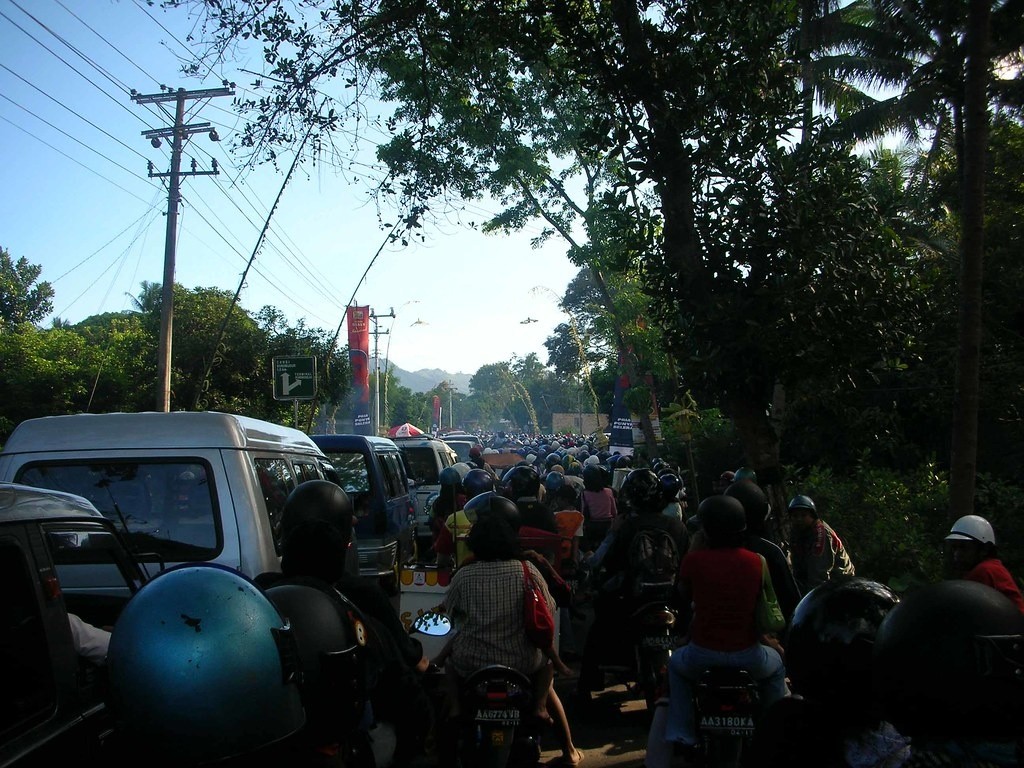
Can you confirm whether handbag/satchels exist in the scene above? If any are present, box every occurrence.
[521,561,555,649]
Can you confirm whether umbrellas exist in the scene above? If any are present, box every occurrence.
[388,422,424,437]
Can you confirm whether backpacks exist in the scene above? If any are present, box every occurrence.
[631,527,681,600]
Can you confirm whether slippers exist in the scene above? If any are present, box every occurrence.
[566,750,586,768]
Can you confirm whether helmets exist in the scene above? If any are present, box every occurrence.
[102,567,308,768]
[729,466,755,482]
[618,467,661,505]
[544,470,566,491]
[462,469,493,497]
[463,491,521,555]
[782,575,902,705]
[509,466,540,498]
[787,494,819,519]
[688,495,749,537]
[661,473,680,498]
[943,514,996,554]
[869,578,1024,738]
[272,477,359,567]
[450,433,687,475]
[438,467,461,486]
[259,581,377,750]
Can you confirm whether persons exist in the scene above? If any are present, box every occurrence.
[0,427,1024,768]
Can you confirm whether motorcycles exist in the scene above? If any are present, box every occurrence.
[592,566,684,730]
[676,665,760,768]
[447,665,553,768]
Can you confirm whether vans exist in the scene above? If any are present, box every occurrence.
[2,409,353,584]
[0,480,151,767]
[442,436,482,464]
[390,435,464,538]
[308,434,427,592]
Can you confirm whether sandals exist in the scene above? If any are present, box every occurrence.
[553,663,575,676]
[536,707,557,726]
